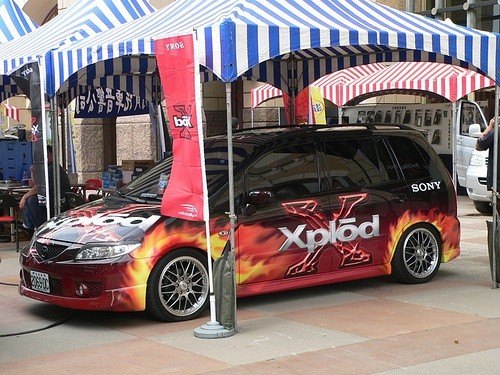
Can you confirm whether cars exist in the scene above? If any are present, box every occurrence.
[16,124,461,322]
[455,100,496,214]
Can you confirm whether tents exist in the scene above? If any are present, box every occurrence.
[0,0,500,122]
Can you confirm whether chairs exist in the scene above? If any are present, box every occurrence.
[0,178,119,253]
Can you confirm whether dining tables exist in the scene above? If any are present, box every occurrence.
[0,182,33,243]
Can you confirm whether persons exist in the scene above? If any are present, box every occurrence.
[476,99,500,221]
[17,145,71,233]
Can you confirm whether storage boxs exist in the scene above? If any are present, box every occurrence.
[102,160,153,192]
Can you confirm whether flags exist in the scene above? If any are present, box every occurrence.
[282,86,327,125]
[153,33,204,221]
